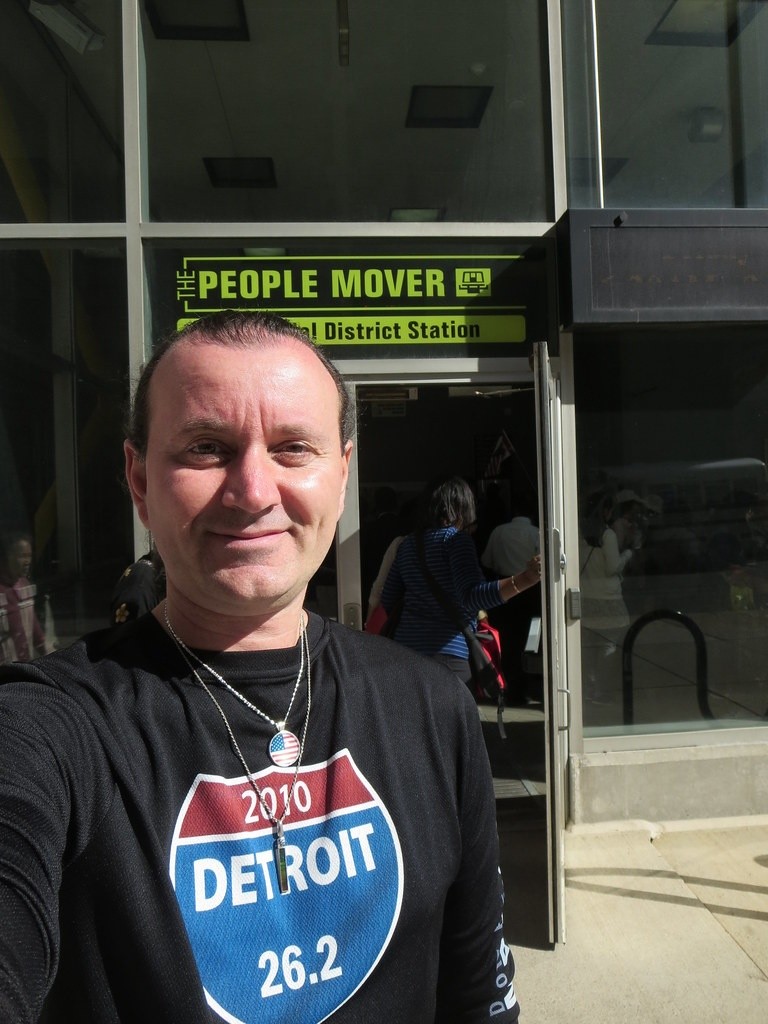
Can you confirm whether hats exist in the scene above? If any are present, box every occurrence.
[616,489,646,504]
[644,494,663,514]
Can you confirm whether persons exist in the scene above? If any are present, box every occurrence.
[580,490,659,706]
[1,312,542,1024]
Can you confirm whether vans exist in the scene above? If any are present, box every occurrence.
[577,457,768,573]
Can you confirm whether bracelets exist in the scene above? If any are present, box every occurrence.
[509,572,519,597]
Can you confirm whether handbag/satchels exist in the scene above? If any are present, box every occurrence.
[468,612,514,740]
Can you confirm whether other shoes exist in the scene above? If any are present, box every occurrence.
[585,692,594,700]
[592,695,617,706]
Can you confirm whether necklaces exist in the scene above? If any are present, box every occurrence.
[164,602,313,769]
[167,626,311,896]
